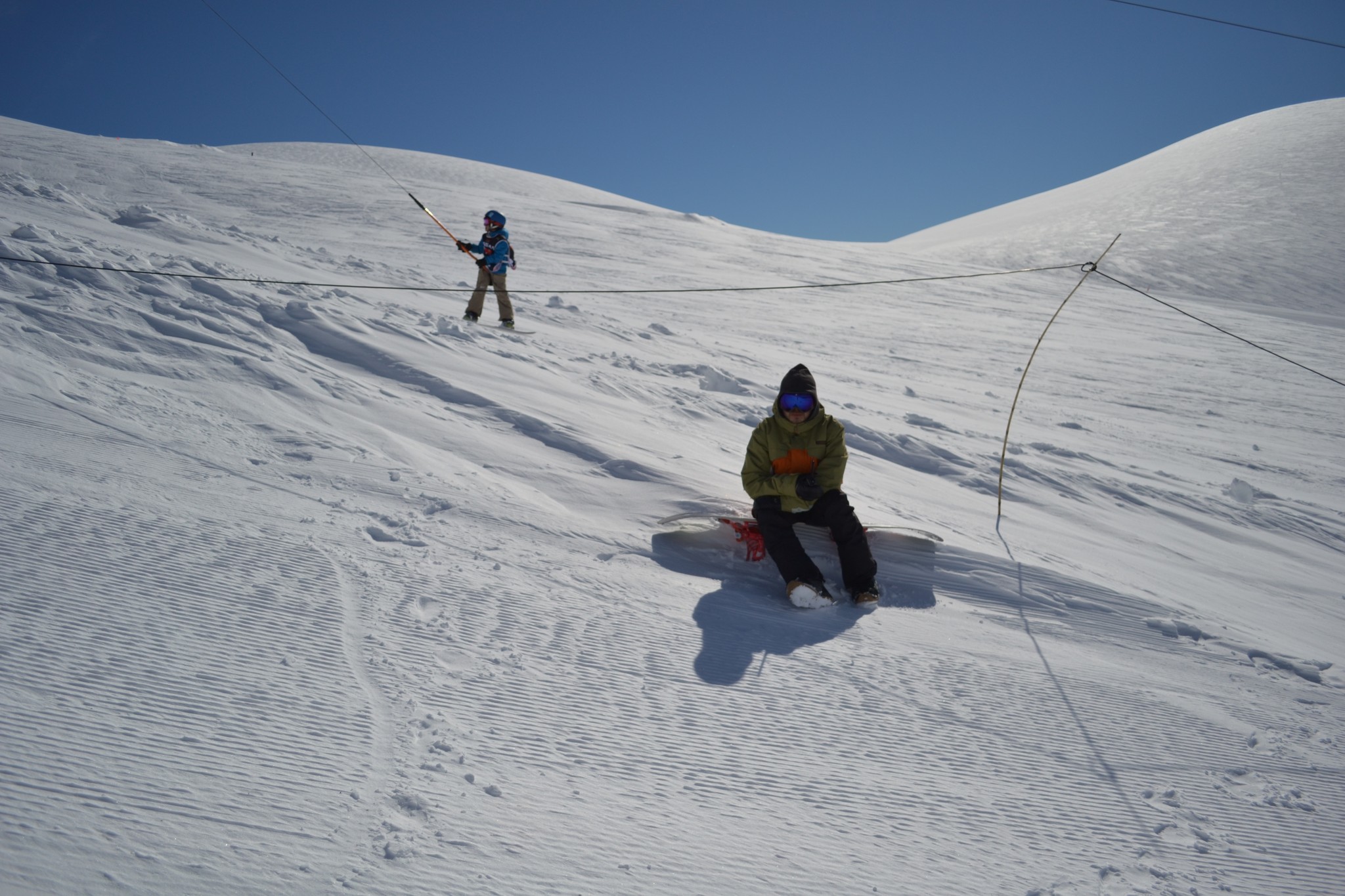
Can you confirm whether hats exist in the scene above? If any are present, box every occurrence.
[778,363,818,401]
[484,210,507,229]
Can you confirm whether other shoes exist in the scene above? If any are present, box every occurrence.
[498,319,516,330]
[463,311,478,323]
[787,576,836,608]
[852,576,882,605]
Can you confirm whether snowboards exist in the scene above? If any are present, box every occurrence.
[658,511,944,542]
[439,312,536,335]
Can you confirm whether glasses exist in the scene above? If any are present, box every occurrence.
[482,217,502,227]
[778,392,816,411]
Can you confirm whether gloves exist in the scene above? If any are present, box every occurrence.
[475,258,487,269]
[795,471,821,502]
[455,239,472,253]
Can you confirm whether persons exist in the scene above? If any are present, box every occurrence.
[741,364,879,609]
[457,211,515,330]
